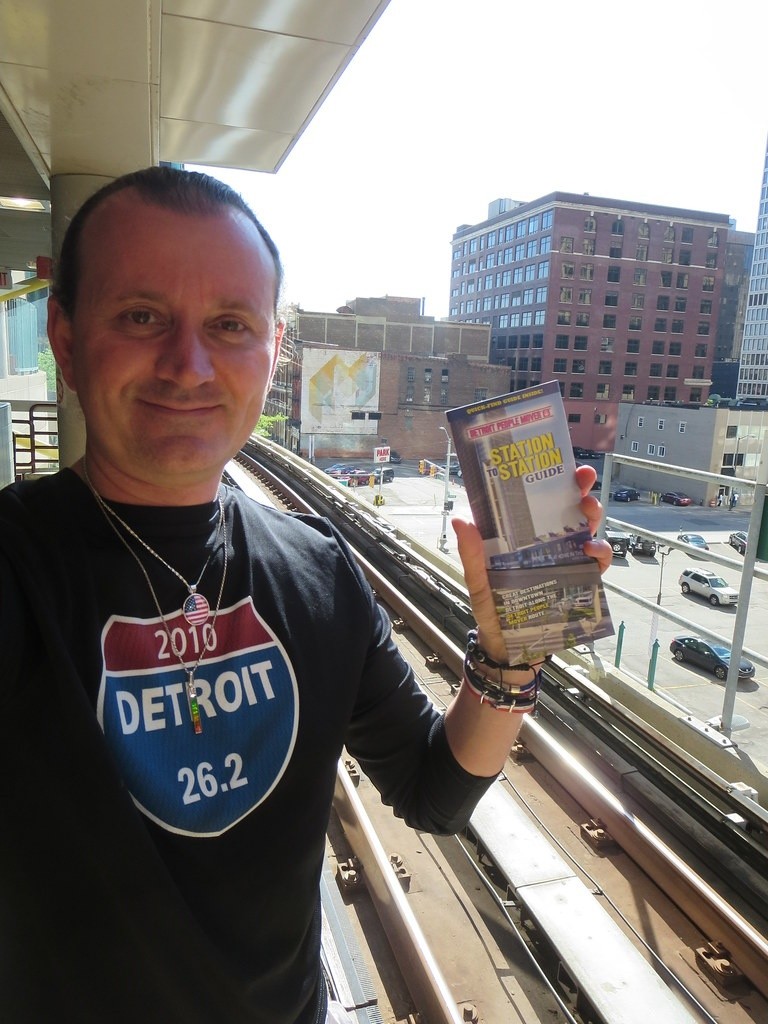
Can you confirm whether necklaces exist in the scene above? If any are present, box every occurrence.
[86,477,228,736]
[83,457,223,625]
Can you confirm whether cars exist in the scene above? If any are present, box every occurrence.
[325,462,370,485]
[441,463,463,478]
[670,636,755,679]
[614,488,641,502]
[626,534,656,557]
[729,531,747,554]
[660,491,691,506]
[677,533,710,557]
[389,450,402,464]
[591,482,602,490]
[572,446,601,459]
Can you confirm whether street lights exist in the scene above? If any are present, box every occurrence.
[438,426,452,553]
[728,434,757,511]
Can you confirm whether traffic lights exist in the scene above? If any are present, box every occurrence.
[429,464,435,477]
[417,459,425,474]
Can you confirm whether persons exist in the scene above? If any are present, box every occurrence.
[733,493,738,507]
[717,492,723,505]
[0,169,611,1024]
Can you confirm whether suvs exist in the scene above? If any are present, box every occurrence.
[605,530,627,559]
[679,567,740,607]
[372,467,394,484]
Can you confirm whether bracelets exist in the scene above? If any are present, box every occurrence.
[462,655,542,694]
[463,658,538,714]
[467,627,552,718]
